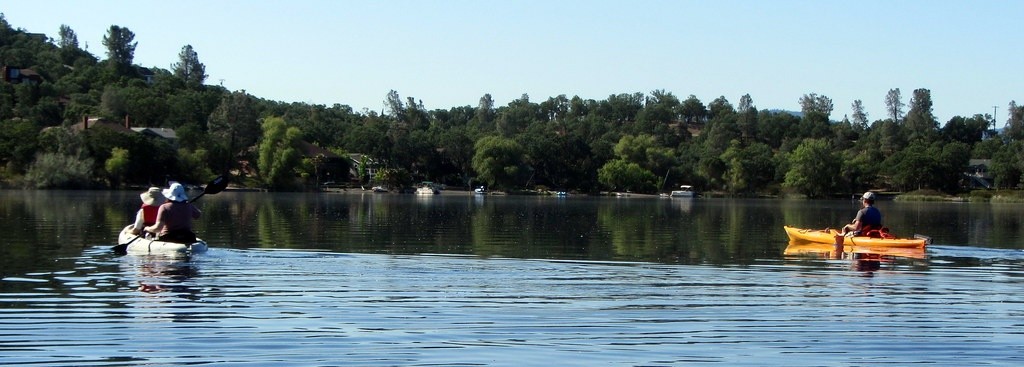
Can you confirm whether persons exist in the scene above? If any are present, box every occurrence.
[125,187,166,239]
[825,192,881,236]
[143,183,201,248]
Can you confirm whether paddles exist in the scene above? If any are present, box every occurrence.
[835,219,856,244]
[111,173,229,254]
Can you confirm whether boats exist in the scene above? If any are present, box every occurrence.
[112,220,211,262]
[784,224,931,250]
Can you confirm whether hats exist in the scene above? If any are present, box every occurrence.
[139,186,165,207]
[860,191,875,200]
[161,182,188,202]
